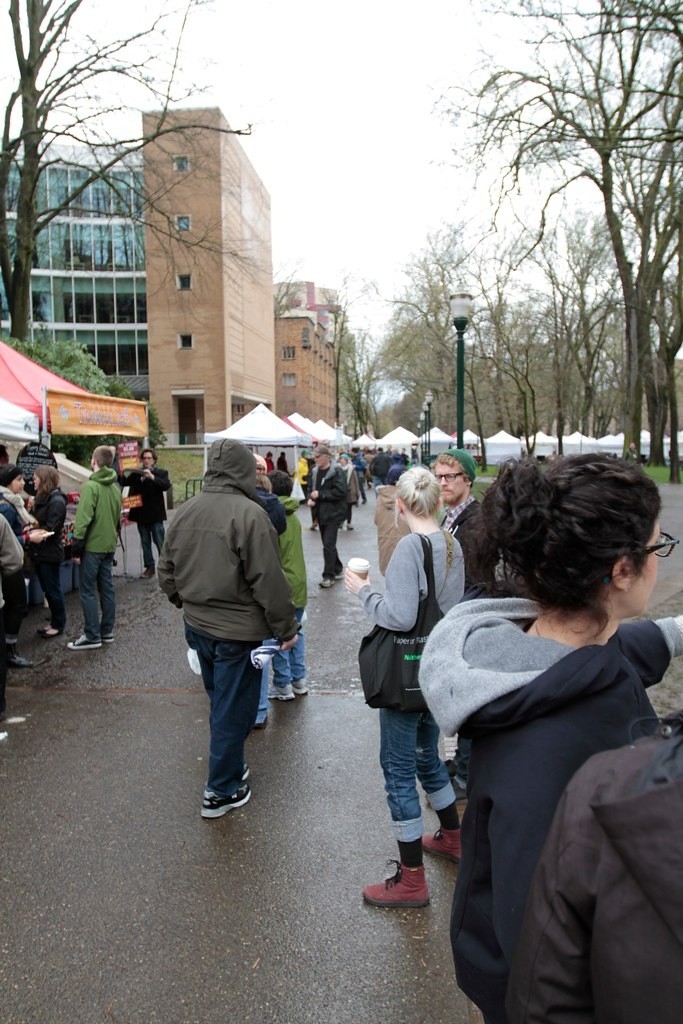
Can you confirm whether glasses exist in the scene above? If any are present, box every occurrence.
[633,531,680,558]
[436,472,464,483]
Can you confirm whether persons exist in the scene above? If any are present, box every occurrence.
[65,445,121,649]
[448,454,683,1024]
[0,513,24,717]
[0,445,8,468]
[156,440,299,819]
[506,709,683,1024]
[249,443,640,909]
[0,464,47,666]
[26,465,66,638]
[118,448,170,578]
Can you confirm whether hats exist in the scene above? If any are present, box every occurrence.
[308,445,331,457]
[442,448,478,482]
[253,453,267,475]
[385,464,404,484]
[340,453,350,461]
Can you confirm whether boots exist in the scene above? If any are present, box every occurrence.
[5,642,34,667]
[362,860,429,908]
[422,826,461,864]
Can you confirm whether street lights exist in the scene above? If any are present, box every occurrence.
[417,401,428,465]
[450,291,475,449]
[426,390,435,467]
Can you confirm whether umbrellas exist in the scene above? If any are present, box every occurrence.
[250,625,302,670]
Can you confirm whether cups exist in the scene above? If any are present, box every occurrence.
[347,558,371,594]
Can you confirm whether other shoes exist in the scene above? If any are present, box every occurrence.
[140,567,155,577]
[425,759,469,806]
[339,521,354,530]
[361,499,367,504]
[334,568,346,579]
[319,577,335,588]
[311,524,317,530]
[37,628,63,638]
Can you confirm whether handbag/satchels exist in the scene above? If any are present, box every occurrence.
[358,532,445,713]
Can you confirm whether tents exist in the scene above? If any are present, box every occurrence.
[0,341,683,590]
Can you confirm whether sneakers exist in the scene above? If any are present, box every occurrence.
[66,634,114,650]
[200,763,251,818]
[268,678,309,700]
